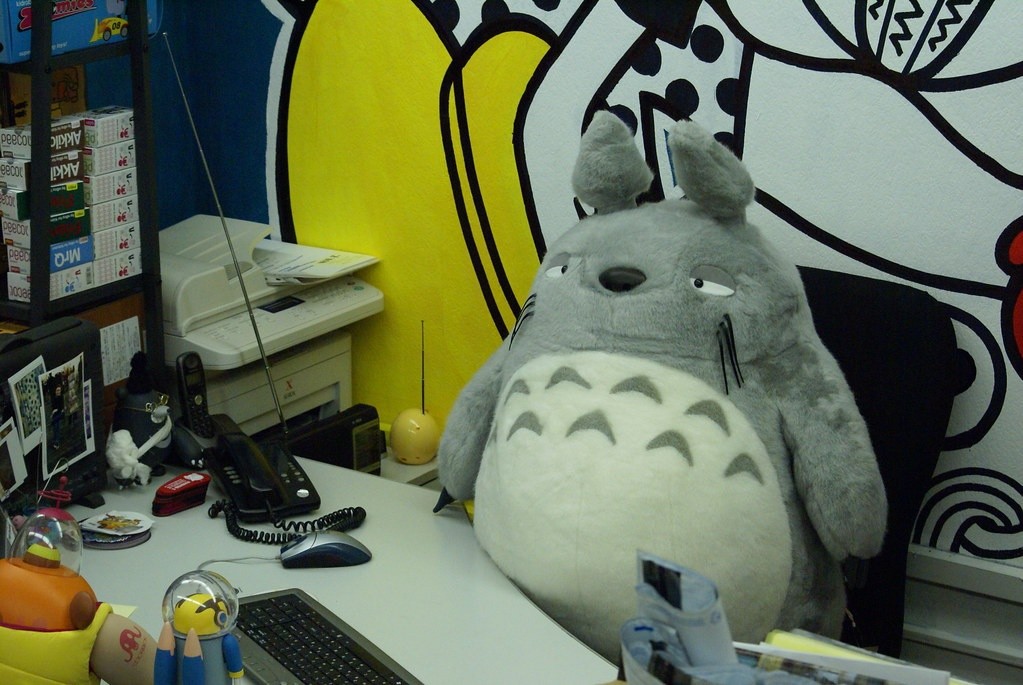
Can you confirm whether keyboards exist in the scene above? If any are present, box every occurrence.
[229,587,425,685]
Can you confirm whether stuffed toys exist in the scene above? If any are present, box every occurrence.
[435,109,889,668]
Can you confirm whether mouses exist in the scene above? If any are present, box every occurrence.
[281,529,373,569]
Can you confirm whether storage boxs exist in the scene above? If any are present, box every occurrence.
[93,248,142,287]
[91,223,141,260]
[7,234,93,274]
[0,148,84,191]
[74,104,134,147]
[0,0,162,65]
[84,167,137,205]
[83,140,136,176]
[88,195,138,232]
[0,115,85,159]
[0,65,86,126]
[0,180,84,221]
[68,296,149,406]
[2,206,91,246]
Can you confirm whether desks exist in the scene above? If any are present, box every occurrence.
[41,457,618,685]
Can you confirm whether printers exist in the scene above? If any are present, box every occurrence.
[158,212,388,441]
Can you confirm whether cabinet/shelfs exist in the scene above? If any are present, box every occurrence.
[0,0,164,393]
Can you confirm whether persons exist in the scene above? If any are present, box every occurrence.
[46,385,65,451]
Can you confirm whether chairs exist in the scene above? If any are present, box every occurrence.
[793,264,959,660]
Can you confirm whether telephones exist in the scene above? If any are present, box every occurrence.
[172,351,247,467]
[206,431,320,524]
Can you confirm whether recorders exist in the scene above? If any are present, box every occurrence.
[155,29,381,477]
